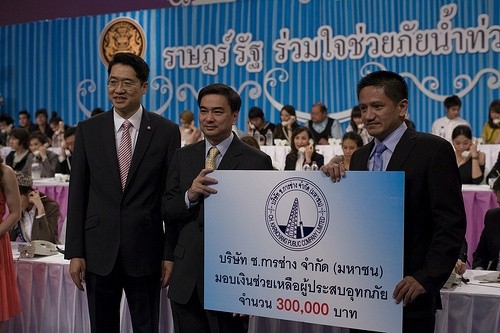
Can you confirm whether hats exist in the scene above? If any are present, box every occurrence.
[14,171,33,186]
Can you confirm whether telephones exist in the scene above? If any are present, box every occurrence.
[1,125,12,133]
[249,121,255,129]
[55,122,64,135]
[358,124,364,129]
[282,116,295,126]
[461,137,478,157]
[299,139,314,153]
[18,240,64,258]
[61,142,71,156]
[493,118,500,125]
[185,120,194,134]
[34,143,49,155]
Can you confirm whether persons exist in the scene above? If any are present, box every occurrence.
[0,107,77,178]
[486,150,500,185]
[178,110,203,147]
[319,71,467,333]
[345,105,375,146]
[329,132,364,172]
[8,171,60,245]
[64,52,181,333]
[452,125,485,185]
[162,84,272,333]
[456,237,468,276]
[247,107,276,146]
[0,154,22,333]
[91,108,104,116]
[272,105,303,146]
[432,95,471,146]
[284,126,324,171]
[480,100,500,144]
[303,103,343,145]
[472,176,500,272]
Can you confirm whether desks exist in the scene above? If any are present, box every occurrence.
[33,178,500,269]
[0,145,500,183]
[0,242,500,333]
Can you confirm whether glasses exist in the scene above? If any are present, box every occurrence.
[107,79,141,91]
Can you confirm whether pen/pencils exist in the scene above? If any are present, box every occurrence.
[496,171,500,176]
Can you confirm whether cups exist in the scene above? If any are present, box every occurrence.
[489,178,497,188]
[55,173,67,183]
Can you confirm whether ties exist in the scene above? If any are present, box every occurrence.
[118,120,132,192]
[205,147,219,169]
[372,143,388,171]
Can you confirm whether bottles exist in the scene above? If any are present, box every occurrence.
[267,129,272,146]
[31,158,40,179]
[254,129,260,145]
[311,161,318,171]
[303,161,310,171]
